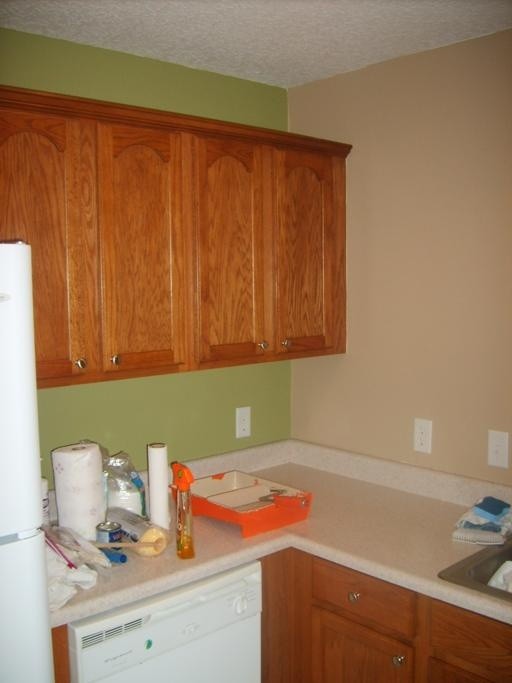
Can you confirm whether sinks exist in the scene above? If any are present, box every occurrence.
[438,536,512,600]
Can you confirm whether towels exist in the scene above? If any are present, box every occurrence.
[452,495,512,544]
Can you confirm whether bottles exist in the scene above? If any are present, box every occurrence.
[95,521,122,551]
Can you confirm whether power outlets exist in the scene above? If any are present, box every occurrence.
[414,418,432,454]
[236,406,251,439]
[488,430,508,468]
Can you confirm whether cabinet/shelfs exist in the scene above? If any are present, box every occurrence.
[0,85,354,389]
[51,438,512,683]
